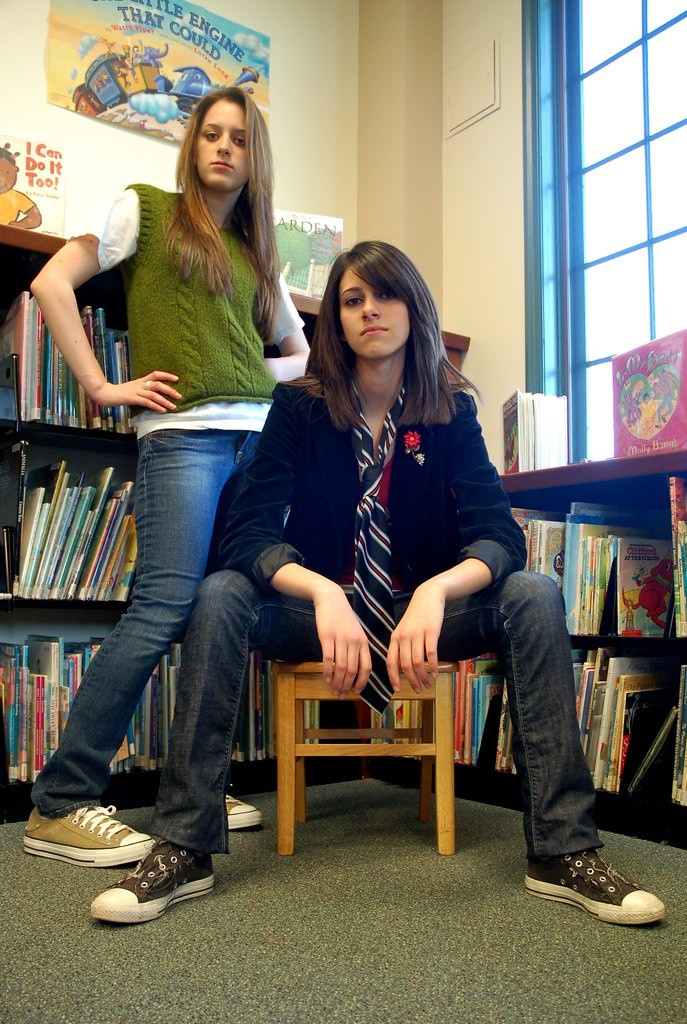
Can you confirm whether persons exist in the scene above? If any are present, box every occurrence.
[87,239,666,927]
[20,84,310,868]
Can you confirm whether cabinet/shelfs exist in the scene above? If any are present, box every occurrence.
[370,448,687,849]
[0,226,472,829]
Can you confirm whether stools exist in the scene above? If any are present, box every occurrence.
[265,658,460,857]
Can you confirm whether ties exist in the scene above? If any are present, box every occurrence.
[346,383,406,714]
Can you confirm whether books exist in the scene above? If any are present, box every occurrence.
[1,292,277,782]
[371,390,686,807]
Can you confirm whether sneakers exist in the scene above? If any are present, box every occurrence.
[23,805,156,867]
[524,848,666,924]
[226,794,263,829]
[89,841,215,923]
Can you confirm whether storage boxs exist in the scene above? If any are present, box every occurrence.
[610,328,687,458]
